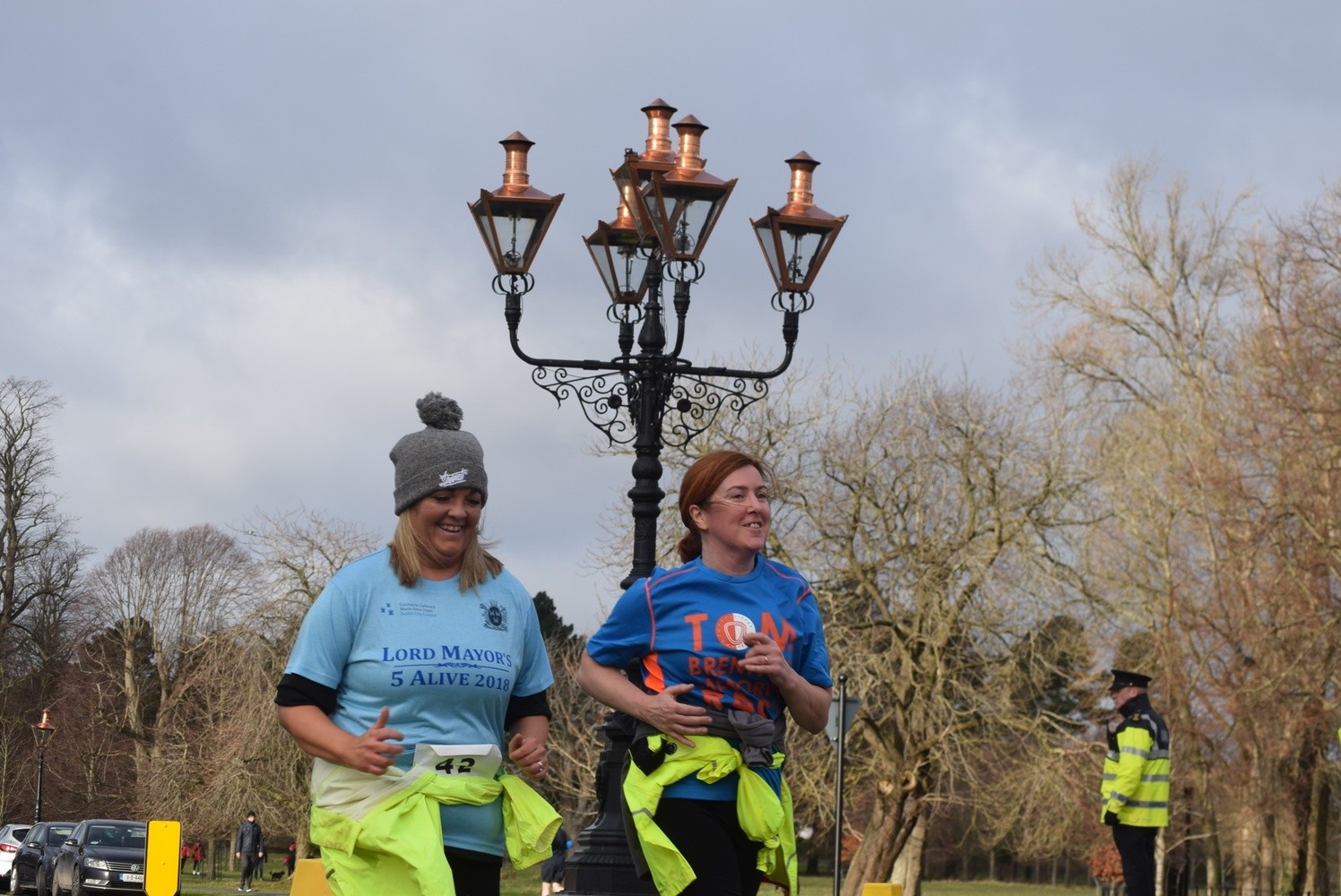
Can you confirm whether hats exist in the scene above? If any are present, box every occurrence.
[385,390,491,514]
[1106,668,1154,691]
[247,810,257,818]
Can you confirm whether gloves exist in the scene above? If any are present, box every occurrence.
[1103,811,1121,828]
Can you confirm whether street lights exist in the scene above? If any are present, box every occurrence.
[31,708,59,822]
[467,97,851,896]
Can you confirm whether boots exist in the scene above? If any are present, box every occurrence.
[541,882,551,896]
[553,882,565,893]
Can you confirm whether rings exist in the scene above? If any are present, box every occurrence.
[764,655,768,665]
[538,761,543,771]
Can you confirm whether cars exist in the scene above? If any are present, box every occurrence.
[0,824,34,892]
[9,821,79,896]
[52,818,146,896]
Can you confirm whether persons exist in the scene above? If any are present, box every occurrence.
[541,829,567,896]
[577,450,832,895]
[236,811,263,892]
[1100,669,1169,896]
[284,845,296,876]
[192,840,205,875]
[254,845,267,880]
[181,840,190,873]
[275,395,563,896]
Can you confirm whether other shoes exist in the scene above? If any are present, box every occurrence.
[198,872,201,875]
[192,870,196,875]
[238,887,243,891]
[245,888,252,893]
